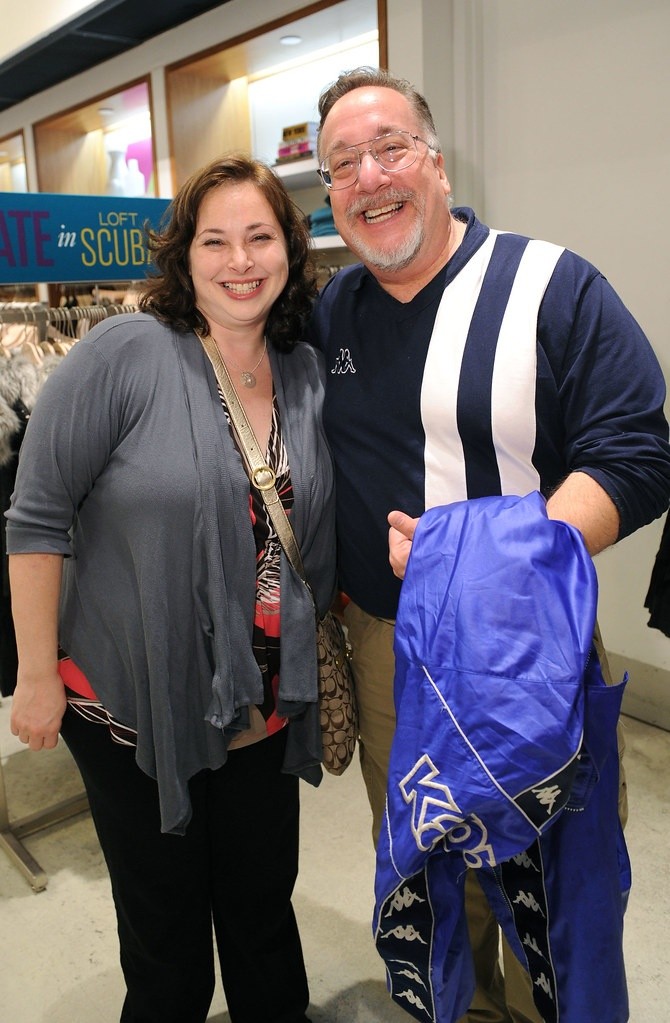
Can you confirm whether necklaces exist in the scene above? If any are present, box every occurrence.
[222,341,266,387]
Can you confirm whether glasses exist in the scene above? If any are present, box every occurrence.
[317,131,438,191]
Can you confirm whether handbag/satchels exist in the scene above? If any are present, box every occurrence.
[315,609,358,776]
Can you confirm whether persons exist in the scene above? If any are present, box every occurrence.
[306,70,670,1023]
[3,157,338,1023]
